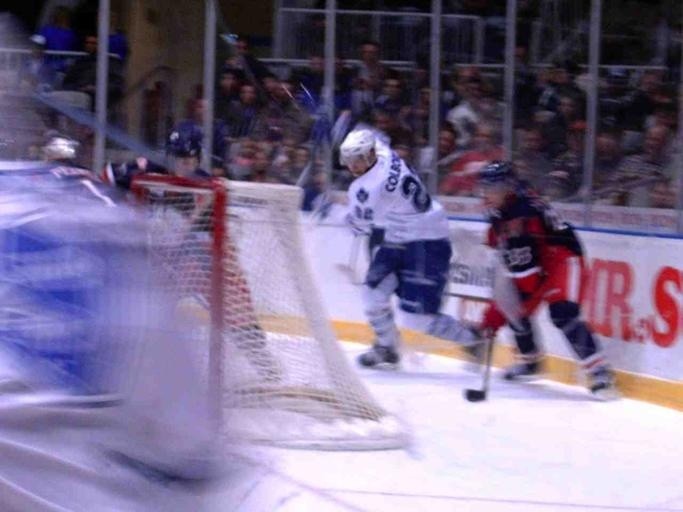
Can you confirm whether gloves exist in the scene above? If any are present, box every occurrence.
[479,306,505,340]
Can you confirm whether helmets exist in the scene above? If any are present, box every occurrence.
[339,130,376,167]
[479,160,517,190]
[168,121,203,158]
[44,138,76,161]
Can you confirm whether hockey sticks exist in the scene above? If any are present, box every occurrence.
[463,326,494,402]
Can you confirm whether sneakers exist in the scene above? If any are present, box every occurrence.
[358,344,400,366]
[590,365,616,393]
[463,323,486,373]
[502,361,536,380]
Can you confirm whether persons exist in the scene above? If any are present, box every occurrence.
[22,132,119,206]
[0,24,257,512]
[470,159,617,392]
[290,106,484,366]
[98,120,282,387]
